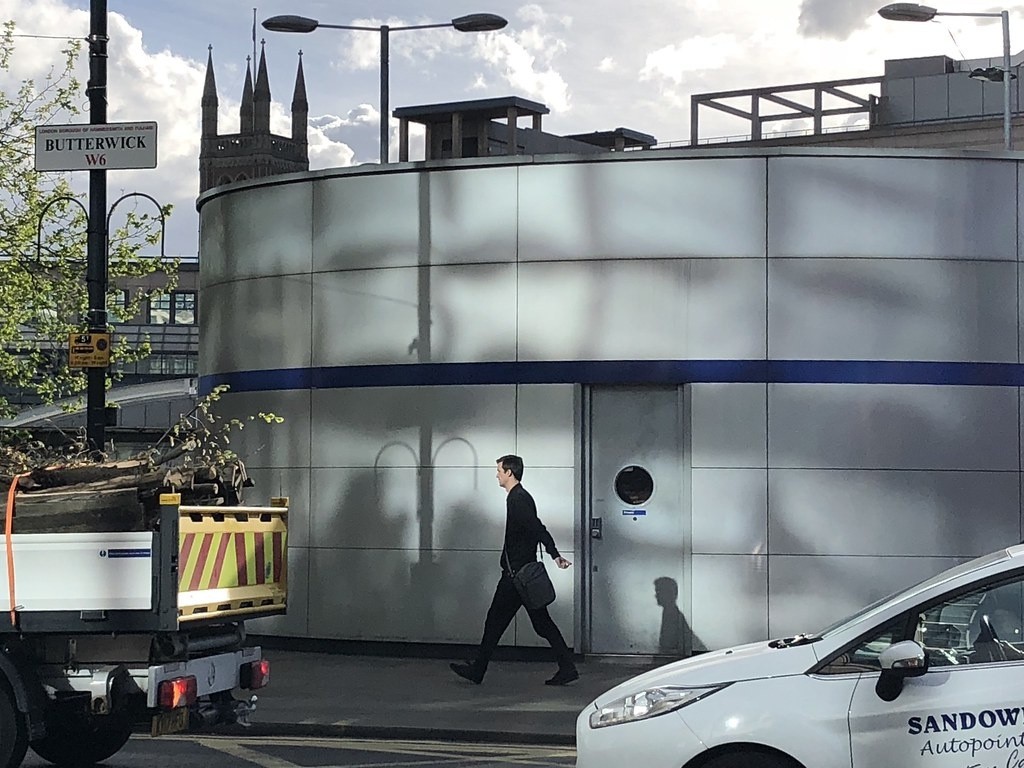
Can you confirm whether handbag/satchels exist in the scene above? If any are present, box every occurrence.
[513,562,556,609]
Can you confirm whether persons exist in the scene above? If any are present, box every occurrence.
[449,455,581,688]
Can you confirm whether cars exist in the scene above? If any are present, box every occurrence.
[576,543,1024,768]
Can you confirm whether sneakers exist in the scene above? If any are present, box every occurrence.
[546,669,581,686]
[449,662,484,685]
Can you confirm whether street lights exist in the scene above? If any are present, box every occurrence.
[879,3,1011,149]
[30,192,175,462]
[262,13,508,165]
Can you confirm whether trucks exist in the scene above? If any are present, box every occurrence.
[0,494,291,767]
